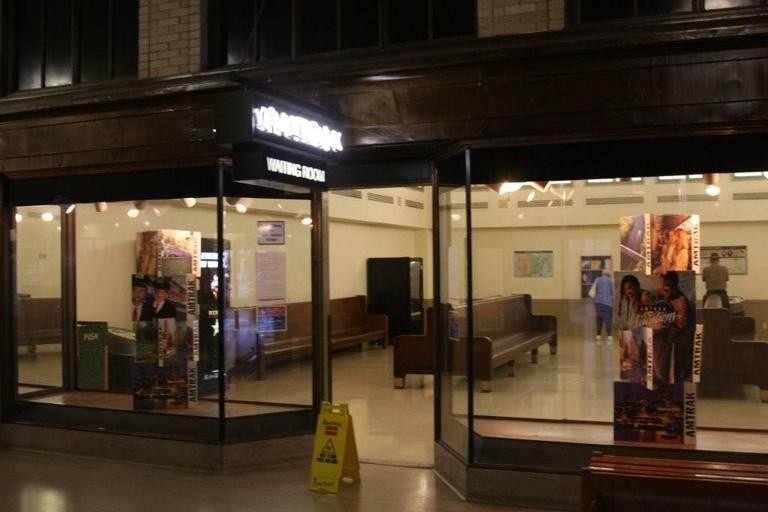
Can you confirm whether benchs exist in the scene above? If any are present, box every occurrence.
[693,303,768,406]
[440,289,561,394]
[388,292,504,392]
[12,291,63,356]
[240,292,391,382]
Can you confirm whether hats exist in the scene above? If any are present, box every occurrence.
[153,279,171,291]
[132,274,152,287]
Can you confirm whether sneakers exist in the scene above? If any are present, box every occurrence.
[595,335,614,344]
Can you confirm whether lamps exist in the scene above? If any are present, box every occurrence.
[54,195,313,228]
[479,172,722,200]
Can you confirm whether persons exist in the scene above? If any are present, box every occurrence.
[149,279,179,317]
[593,269,613,340]
[132,274,155,319]
[648,272,691,382]
[614,275,651,375]
[702,252,732,308]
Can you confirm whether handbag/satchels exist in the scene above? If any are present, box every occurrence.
[587,280,598,299]
